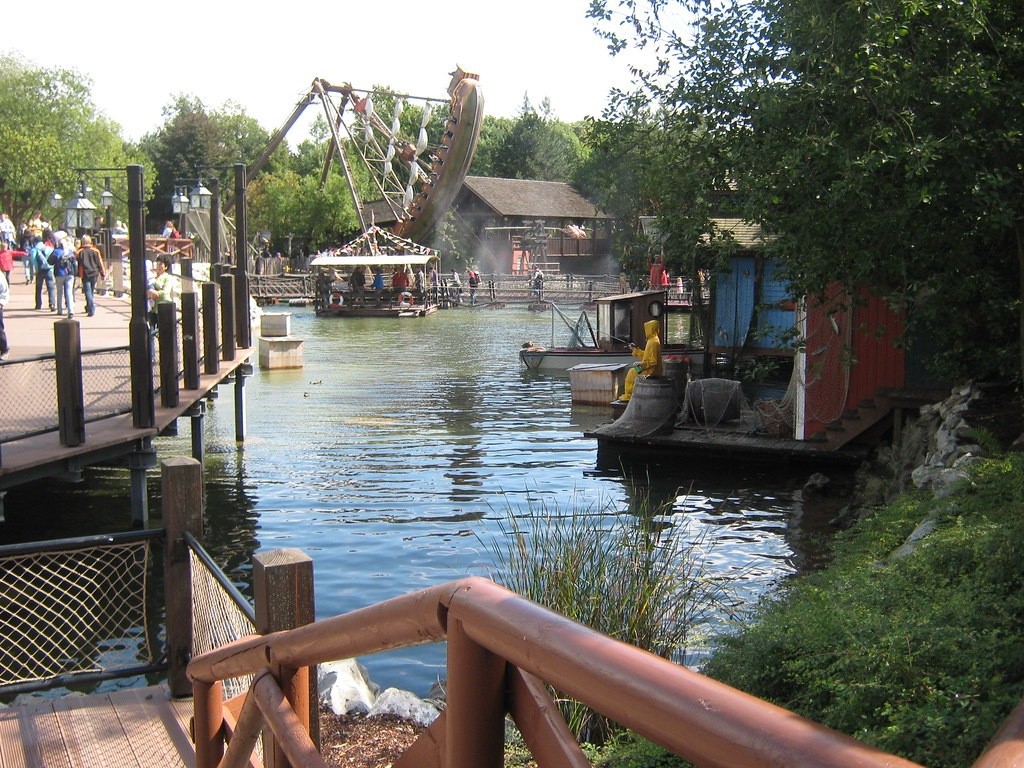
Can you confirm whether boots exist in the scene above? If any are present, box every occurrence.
[618,379,634,402]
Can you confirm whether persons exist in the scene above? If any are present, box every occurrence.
[18,210,105,318]
[274,249,282,263]
[698,269,712,292]
[619,320,662,402]
[449,268,461,298]
[112,221,128,275]
[0,269,8,360]
[677,278,694,305]
[0,214,16,251]
[467,269,478,305]
[162,221,176,263]
[533,265,542,299]
[620,272,651,294]
[316,265,437,313]
[145,255,173,367]
[0,242,28,290]
[262,245,272,258]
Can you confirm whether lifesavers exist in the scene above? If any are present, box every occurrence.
[398,292,413,307]
[329,292,344,308]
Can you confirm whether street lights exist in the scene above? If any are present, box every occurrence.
[171,162,253,350]
[52,177,116,296]
[66,164,157,428]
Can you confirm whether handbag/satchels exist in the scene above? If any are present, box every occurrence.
[59,255,68,268]
[34,246,52,271]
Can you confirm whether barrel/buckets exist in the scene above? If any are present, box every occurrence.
[661,360,688,413]
[689,378,741,422]
[632,374,676,437]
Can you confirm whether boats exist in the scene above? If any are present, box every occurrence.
[316,228,442,317]
[519,288,709,380]
[398,66,485,247]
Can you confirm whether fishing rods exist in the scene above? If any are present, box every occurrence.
[546,301,640,349]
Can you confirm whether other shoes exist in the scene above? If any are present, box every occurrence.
[50,304,55,311]
[85,305,88,313]
[26,280,29,285]
[29,280,33,284]
[0,350,9,360]
[88,313,93,316]
[35,307,40,309]
[68,314,73,319]
[58,312,62,315]
